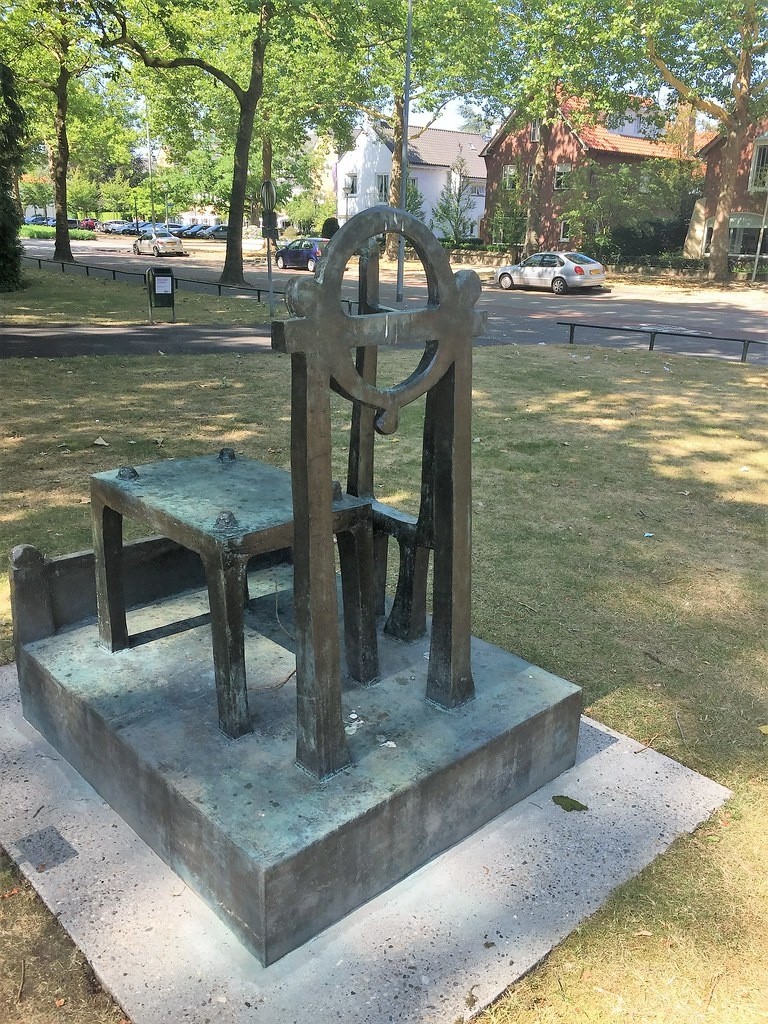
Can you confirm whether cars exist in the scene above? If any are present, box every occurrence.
[27,215,80,228]
[275,238,332,272]
[82,218,164,234]
[133,232,183,257]
[162,223,229,240]
[495,251,605,294]
